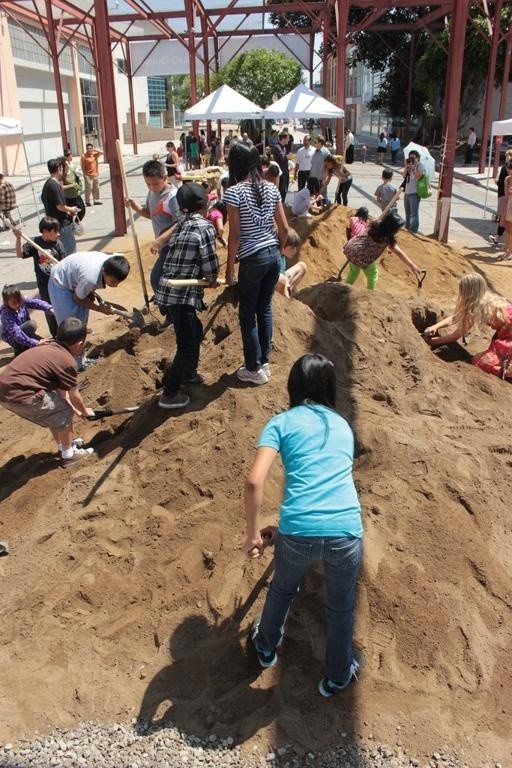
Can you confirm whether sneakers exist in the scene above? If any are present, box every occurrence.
[157,318,173,330]
[58,438,94,468]
[184,372,204,385]
[250,615,278,668]
[318,649,366,699]
[158,393,191,409]
[84,201,104,207]
[237,363,271,385]
[489,234,512,261]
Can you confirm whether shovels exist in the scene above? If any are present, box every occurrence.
[3,218,128,311]
[325,187,404,281]
[112,307,146,333]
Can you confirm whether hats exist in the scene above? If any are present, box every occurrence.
[175,181,208,213]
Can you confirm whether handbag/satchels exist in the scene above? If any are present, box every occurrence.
[417,174,433,198]
[72,214,86,238]
[175,162,184,181]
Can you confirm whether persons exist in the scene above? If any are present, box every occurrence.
[57,156,86,235]
[0,173,18,231]
[244,353,364,699]
[12,216,66,338]
[64,149,82,175]
[424,272,512,381]
[0,317,96,468]
[80,143,104,206]
[489,145,512,261]
[47,250,130,372]
[464,127,477,164]
[41,158,78,255]
[0,283,54,356]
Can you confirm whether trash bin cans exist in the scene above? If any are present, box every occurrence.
[354,143,366,163]
